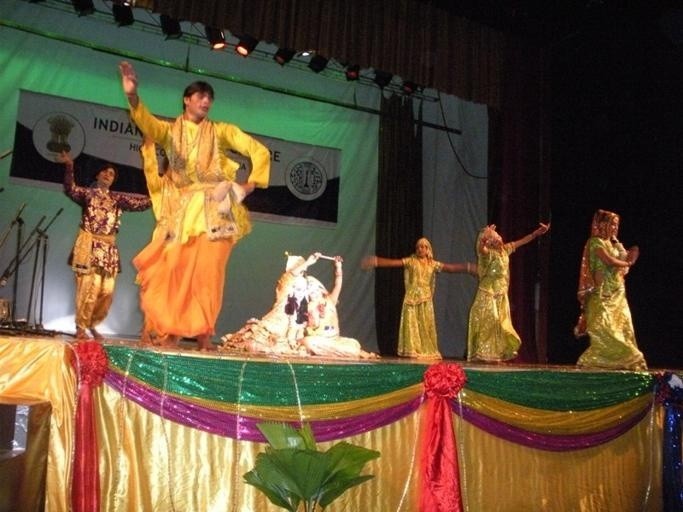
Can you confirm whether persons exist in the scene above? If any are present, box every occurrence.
[49,145,150,340]
[219,252,322,356]
[465,219,550,365]
[303,255,383,360]
[139,133,170,225]
[571,208,650,371]
[363,234,478,362]
[117,55,271,353]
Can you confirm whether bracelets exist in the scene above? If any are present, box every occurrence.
[374,255,379,268]
[467,261,471,275]
[301,264,306,271]
[626,259,631,266]
[334,271,344,276]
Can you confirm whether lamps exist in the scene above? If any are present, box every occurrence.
[71,1,419,96]
